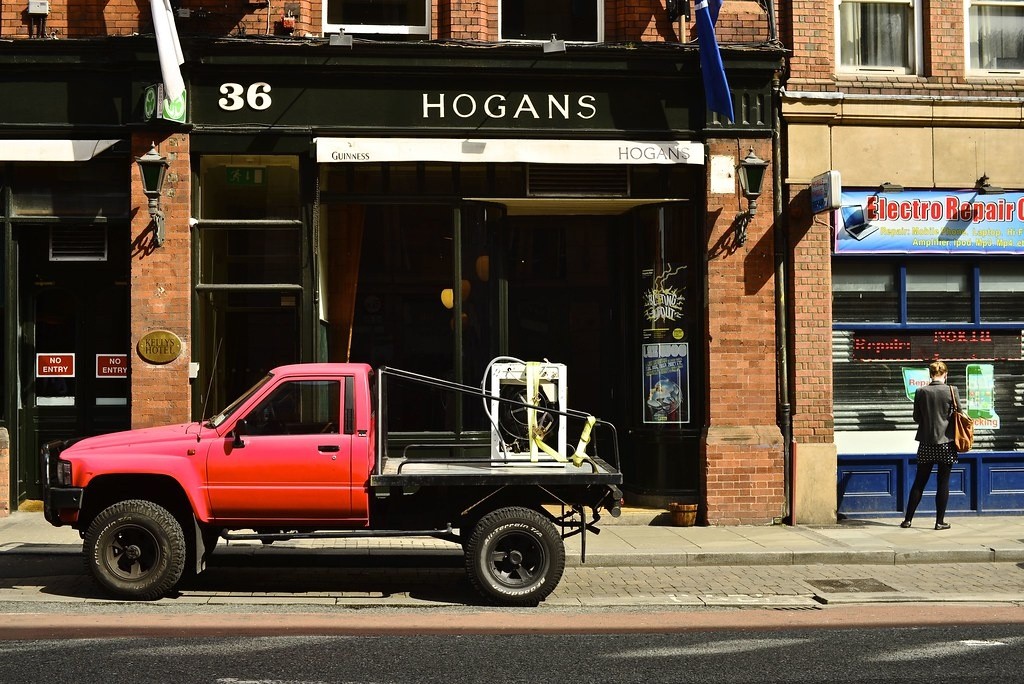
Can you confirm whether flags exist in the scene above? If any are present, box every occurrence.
[696,0,735,124]
[151,0,186,104]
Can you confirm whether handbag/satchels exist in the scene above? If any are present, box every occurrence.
[950,385,974,451]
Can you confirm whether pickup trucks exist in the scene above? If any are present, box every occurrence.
[43,362,623,606]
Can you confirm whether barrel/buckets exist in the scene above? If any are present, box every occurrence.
[668,502,698,527]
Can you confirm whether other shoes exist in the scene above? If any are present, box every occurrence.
[935,522,950,529]
[901,520,911,528]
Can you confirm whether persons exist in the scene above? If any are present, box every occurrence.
[901,362,962,530]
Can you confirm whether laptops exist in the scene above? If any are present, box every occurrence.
[841,204,880,241]
